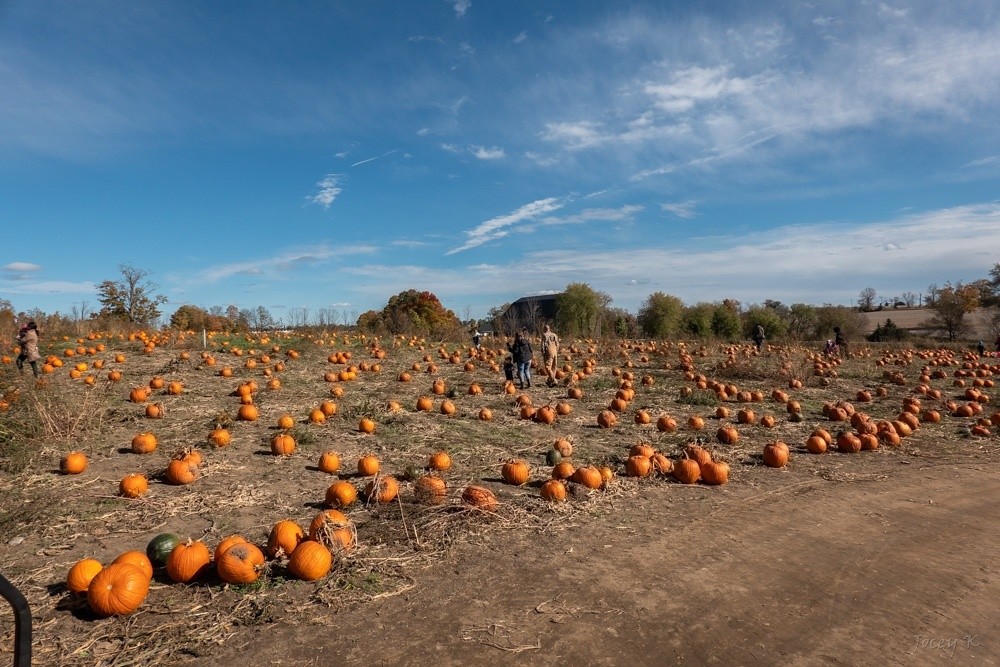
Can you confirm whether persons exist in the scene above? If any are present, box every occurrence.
[542,325,559,388]
[472,329,481,347]
[752,322,765,352]
[19,324,28,356]
[833,326,850,360]
[504,357,514,381]
[16,322,39,378]
[507,330,533,389]
[977,341,985,356]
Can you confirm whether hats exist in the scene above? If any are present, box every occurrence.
[505,357,512,360]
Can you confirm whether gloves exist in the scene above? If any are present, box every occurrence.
[506,342,511,347]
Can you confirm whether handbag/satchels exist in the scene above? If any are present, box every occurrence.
[27,349,41,362]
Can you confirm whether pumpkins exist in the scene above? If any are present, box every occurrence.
[267,519,304,558]
[111,550,153,580]
[146,532,181,567]
[214,534,264,585]
[41,335,1000,511]
[0,347,21,408]
[288,540,331,582]
[67,559,103,592]
[166,537,210,584]
[88,563,149,616]
[309,509,352,554]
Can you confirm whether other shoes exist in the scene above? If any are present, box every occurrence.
[546,382,550,385]
[520,386,524,389]
[528,384,531,387]
[549,383,556,388]
